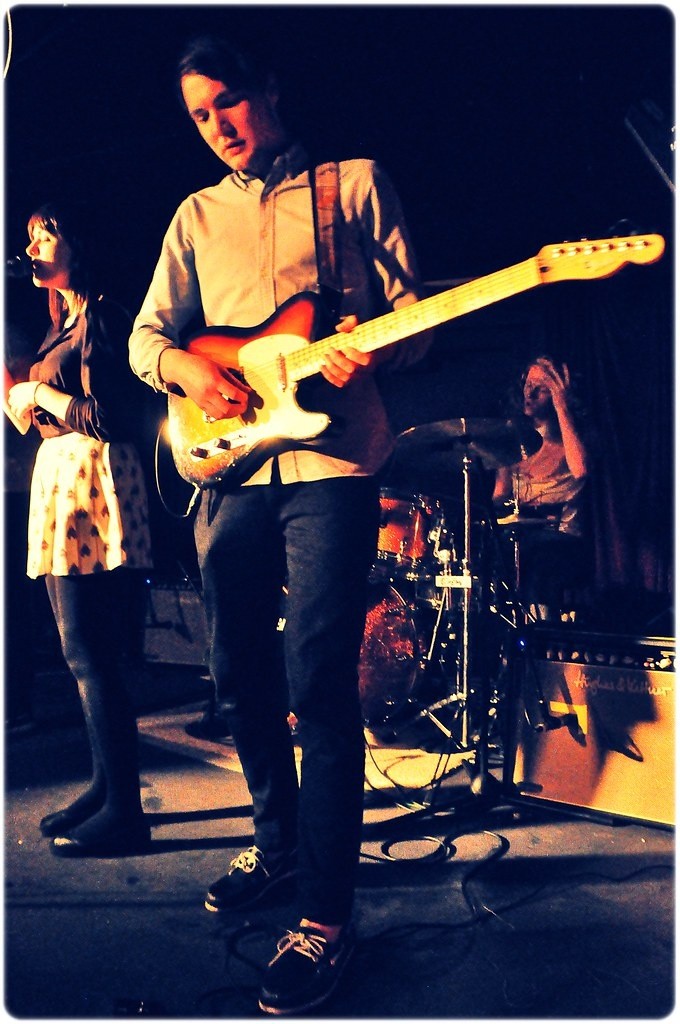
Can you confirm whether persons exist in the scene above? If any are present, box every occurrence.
[9,204,151,854]
[492,356,592,543]
[128,46,432,1013]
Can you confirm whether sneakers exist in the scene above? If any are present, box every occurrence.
[256,924,357,1014]
[204,843,299,914]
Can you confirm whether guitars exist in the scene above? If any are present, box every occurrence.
[165,231,668,497]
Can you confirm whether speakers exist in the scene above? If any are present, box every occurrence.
[510,624,675,834]
[143,584,212,666]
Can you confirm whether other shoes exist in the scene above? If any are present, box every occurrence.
[40,800,76,838]
[50,830,152,858]
[186,720,236,747]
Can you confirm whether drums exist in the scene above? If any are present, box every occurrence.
[372,484,440,568]
[416,552,488,608]
[360,575,472,734]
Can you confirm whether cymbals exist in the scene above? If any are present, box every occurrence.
[399,417,544,474]
[476,514,564,532]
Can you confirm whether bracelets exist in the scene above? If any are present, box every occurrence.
[34,382,44,404]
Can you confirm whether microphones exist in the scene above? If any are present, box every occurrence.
[535,714,577,733]
[145,620,173,630]
[5,255,34,278]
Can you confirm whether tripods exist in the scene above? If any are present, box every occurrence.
[363,455,622,861]
